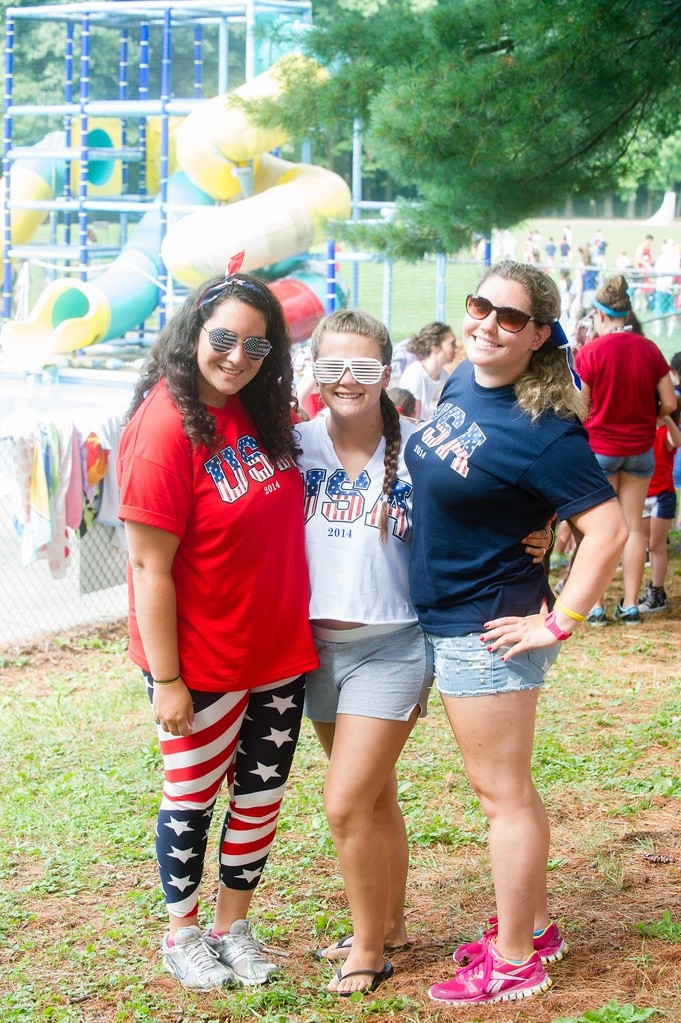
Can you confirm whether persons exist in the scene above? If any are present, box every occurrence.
[117,250,320,993]
[523,224,608,345]
[397,322,458,421]
[405,259,630,1007]
[615,236,681,338]
[289,309,558,997]
[385,388,417,419]
[552,273,681,628]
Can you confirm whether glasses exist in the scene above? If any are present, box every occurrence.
[466,293,540,333]
[200,323,272,362]
[312,357,388,384]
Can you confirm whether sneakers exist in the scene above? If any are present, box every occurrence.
[203,919,278,985]
[638,580,666,613]
[587,608,607,627]
[612,600,640,626]
[427,939,553,1004]
[452,915,569,965]
[161,926,236,993]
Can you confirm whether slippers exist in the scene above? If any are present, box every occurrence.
[314,934,412,963]
[327,963,393,997]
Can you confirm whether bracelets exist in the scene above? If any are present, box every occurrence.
[555,599,586,623]
[547,528,555,550]
[152,676,181,685]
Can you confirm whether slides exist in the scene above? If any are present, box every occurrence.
[0,50,349,370]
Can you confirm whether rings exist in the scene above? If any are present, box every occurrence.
[543,529,548,538]
[542,547,546,556]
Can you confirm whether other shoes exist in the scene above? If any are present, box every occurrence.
[549,551,569,570]
[556,578,565,594]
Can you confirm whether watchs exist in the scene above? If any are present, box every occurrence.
[544,611,572,641]
